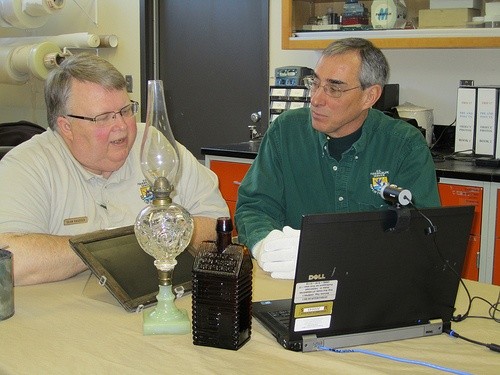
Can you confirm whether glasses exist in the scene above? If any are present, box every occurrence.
[66,100,140,128]
[303,77,362,98]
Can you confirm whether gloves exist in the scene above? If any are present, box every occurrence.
[251,226,301,279]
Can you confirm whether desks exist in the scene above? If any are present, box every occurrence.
[0,236,500,375]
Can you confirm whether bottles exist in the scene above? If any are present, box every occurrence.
[190,216,253,351]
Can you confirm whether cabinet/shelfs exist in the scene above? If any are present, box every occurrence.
[280,0,500,51]
[199,145,500,286]
[270,83,400,125]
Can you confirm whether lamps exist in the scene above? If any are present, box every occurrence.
[134,78,195,340]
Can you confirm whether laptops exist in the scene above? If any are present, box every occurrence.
[250,205,475,352]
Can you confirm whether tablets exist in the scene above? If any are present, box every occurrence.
[68,224,197,312]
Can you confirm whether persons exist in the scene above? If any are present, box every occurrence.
[0,52,231,287]
[235,37,441,279]
